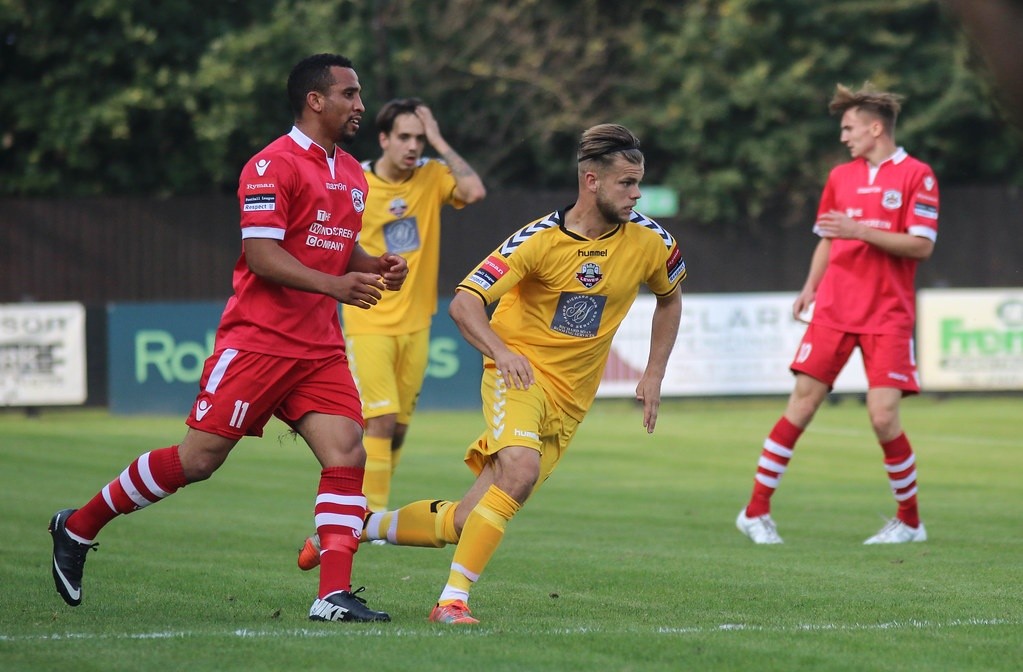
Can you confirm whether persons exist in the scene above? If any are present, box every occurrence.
[734,79,941,546]
[297,123,689,625]
[46,52,410,625]
[341,95,488,548]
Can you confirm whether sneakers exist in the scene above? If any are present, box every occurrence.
[862,514,927,545]
[735,507,782,544]
[298,504,375,571]
[429,599,480,624]
[48,509,99,606]
[309,585,391,623]
